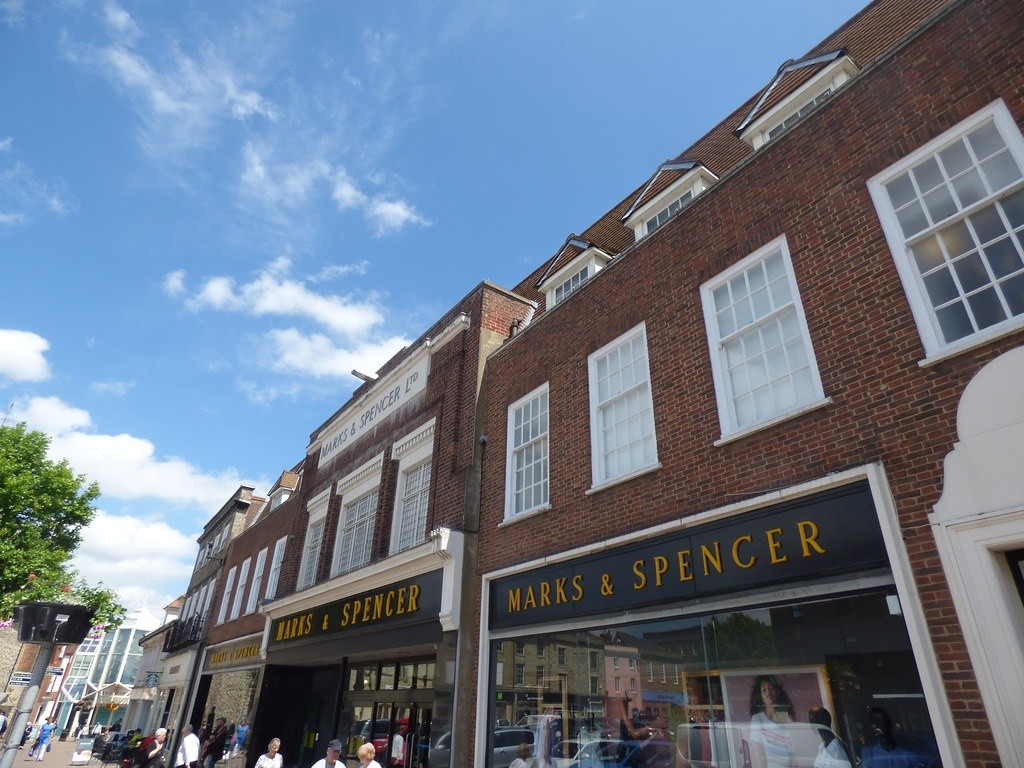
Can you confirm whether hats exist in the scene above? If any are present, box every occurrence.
[329,740,342,751]
[217,717,226,723]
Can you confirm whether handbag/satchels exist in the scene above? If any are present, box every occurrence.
[46,741,52,752]
[29,739,39,756]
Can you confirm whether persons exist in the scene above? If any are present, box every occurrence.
[223,720,249,759]
[750,675,798,768]
[174,724,199,768]
[809,708,852,768]
[101,728,143,762]
[32,718,57,761]
[861,707,920,768]
[392,727,403,764]
[202,717,226,768]
[509,743,531,768]
[91,723,102,747]
[311,739,347,768]
[358,742,382,768]
[109,719,122,732]
[0,711,7,731]
[18,722,32,749]
[137,729,157,763]
[255,738,284,768]
[147,727,167,768]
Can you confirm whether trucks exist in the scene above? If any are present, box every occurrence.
[675,723,862,768]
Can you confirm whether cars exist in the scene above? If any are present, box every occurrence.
[525,739,629,768]
[514,715,561,729]
[569,740,676,768]
[100,733,127,761]
[347,719,400,754]
[623,720,670,740]
[92,732,118,753]
[689,713,719,723]
[428,727,536,768]
[544,717,615,746]
